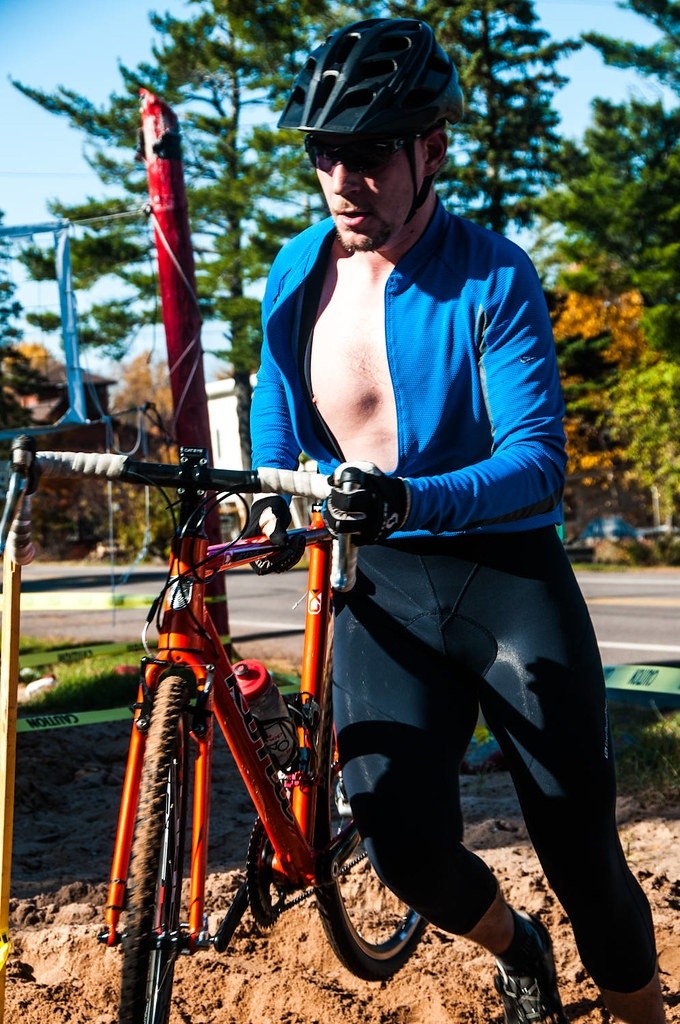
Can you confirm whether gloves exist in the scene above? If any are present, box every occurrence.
[242,494,306,576]
[322,462,408,547]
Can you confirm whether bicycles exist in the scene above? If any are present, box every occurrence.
[4,431,439,1024]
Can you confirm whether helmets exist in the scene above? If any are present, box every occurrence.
[276,18,468,136]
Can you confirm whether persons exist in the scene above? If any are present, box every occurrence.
[242,20,666,1024]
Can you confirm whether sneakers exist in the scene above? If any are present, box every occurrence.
[491,911,568,1024]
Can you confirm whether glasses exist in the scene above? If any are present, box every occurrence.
[304,131,425,173]
